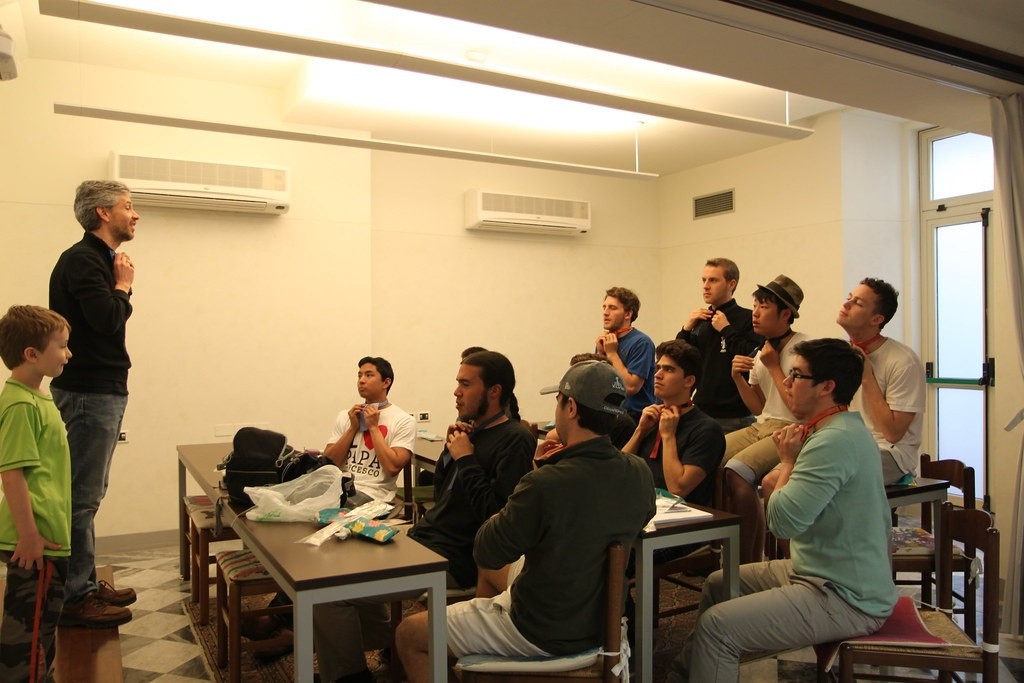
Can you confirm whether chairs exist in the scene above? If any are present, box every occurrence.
[183,456,1000,682]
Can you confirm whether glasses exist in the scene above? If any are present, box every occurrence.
[788,368,815,382]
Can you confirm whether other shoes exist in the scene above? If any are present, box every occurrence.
[376,648,391,664]
[225,608,294,662]
[333,668,376,683]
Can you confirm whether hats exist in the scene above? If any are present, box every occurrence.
[757,275,804,318]
[540,360,627,419]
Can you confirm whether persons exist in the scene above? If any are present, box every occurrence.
[239,355,416,666]
[49,181,140,627]
[668,337,897,683]
[0,305,72,683]
[394,359,657,683]
[312,346,536,683]
[534,257,927,569]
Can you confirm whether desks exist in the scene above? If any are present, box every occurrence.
[405,440,740,682]
[176,443,451,681]
[533,421,950,609]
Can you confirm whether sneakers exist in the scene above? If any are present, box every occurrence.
[42,591,134,627]
[95,579,137,607]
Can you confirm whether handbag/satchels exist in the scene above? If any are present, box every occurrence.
[215,427,357,534]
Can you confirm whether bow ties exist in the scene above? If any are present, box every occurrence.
[748,328,792,358]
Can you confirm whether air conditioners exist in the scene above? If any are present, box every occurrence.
[109,151,288,214]
[463,188,592,235]
